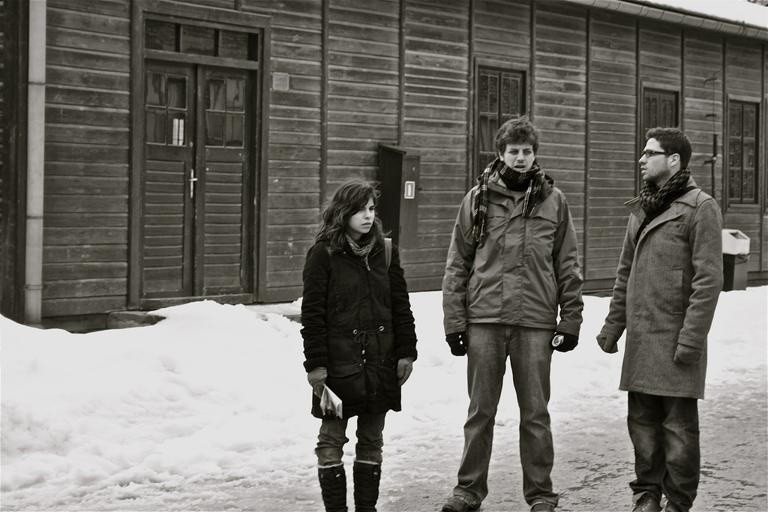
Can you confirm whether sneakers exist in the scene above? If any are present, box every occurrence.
[629,496,663,512]
[442,496,482,512]
[531,503,555,512]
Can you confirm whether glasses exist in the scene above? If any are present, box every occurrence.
[640,149,668,159]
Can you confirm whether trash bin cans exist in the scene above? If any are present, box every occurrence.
[722,229,751,291]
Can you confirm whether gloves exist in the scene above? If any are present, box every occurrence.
[549,333,578,353]
[596,332,620,355]
[307,365,329,399]
[395,357,421,384]
[671,344,705,366]
[446,331,468,357]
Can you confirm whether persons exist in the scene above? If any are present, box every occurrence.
[439,113,584,512]
[595,124,726,512]
[296,178,417,512]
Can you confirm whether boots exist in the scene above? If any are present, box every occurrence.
[317,461,348,511]
[353,459,381,511]
